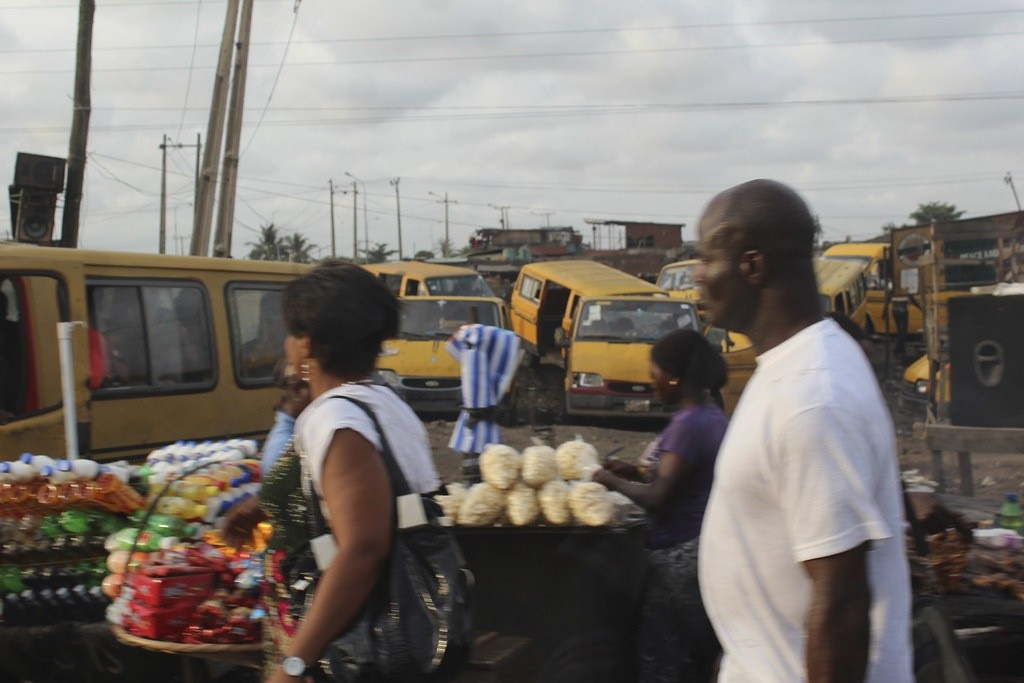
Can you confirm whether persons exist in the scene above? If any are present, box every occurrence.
[593,328,729,683]
[695,180,916,683]
[101,297,208,389]
[262,356,310,478]
[259,258,443,683]
[0,292,19,412]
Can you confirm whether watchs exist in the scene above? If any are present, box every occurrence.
[271,650,310,677]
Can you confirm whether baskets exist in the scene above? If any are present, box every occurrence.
[109,461,269,668]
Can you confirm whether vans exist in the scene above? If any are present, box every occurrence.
[376,301,510,417]
[655,242,997,415]
[0,245,291,465]
[509,258,700,423]
[361,261,496,299]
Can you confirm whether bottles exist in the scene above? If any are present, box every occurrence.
[997,493,1024,533]
[0,436,270,642]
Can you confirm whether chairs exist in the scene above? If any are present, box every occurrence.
[616,317,633,331]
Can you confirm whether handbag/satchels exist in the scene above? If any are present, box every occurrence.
[909,592,977,683]
[281,395,475,683]
